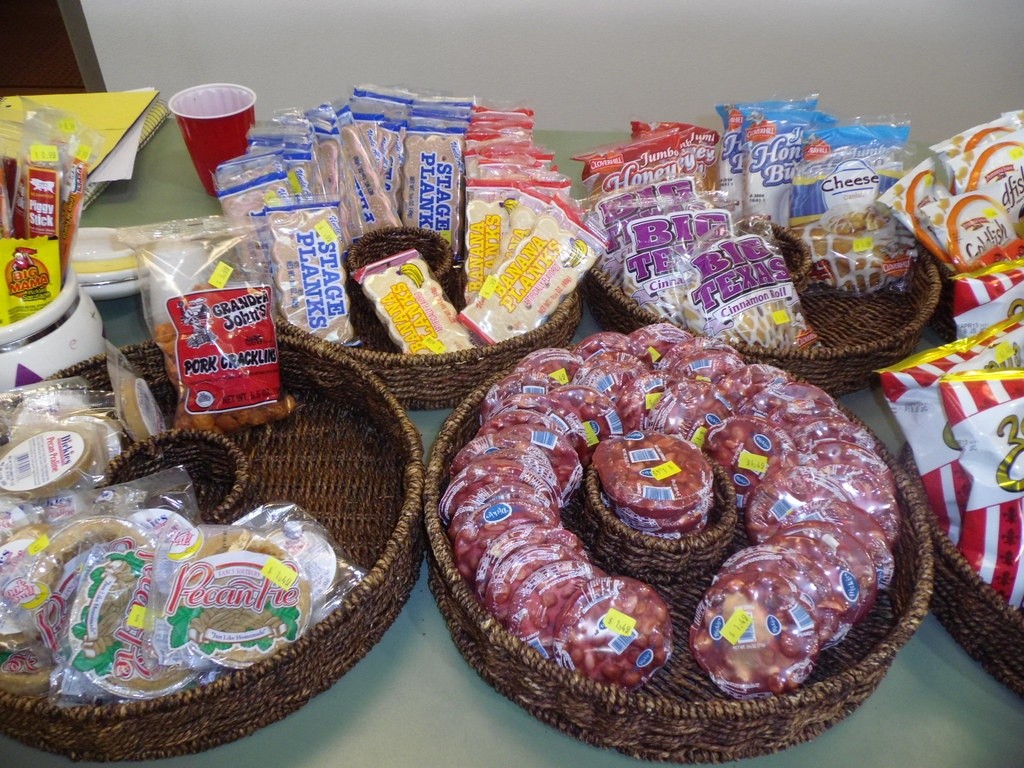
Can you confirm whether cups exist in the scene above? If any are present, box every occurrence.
[168,83,257,197]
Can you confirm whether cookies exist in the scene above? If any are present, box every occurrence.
[0,371,353,703]
[211,83,607,358]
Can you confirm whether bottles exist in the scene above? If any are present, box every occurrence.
[0,267,106,393]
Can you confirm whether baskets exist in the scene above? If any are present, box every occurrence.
[274,226,581,409]
[934,280,958,340]
[583,211,940,398]
[0,335,425,760]
[899,442,1024,696]
[425,362,934,762]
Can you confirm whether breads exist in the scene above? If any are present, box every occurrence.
[559,88,915,352]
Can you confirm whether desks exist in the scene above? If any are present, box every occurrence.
[0,115,1024,768]
